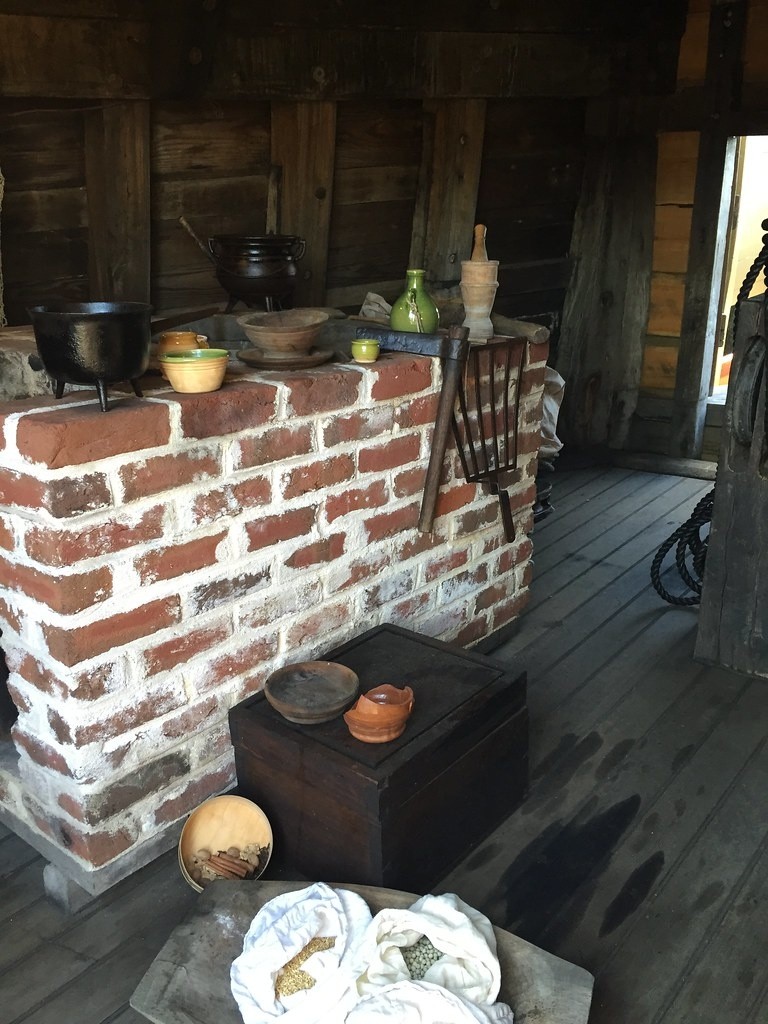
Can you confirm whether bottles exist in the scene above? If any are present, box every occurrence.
[389,269,439,335]
[158,331,199,381]
[196,335,210,349]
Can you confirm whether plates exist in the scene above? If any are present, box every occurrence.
[236,345,333,370]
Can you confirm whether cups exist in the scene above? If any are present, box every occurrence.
[350,339,381,363]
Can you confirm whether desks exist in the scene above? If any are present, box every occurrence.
[227,623,528,892]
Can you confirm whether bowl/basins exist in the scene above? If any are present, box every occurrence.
[158,355,229,394]
[158,349,229,362]
[178,795,274,893]
[264,660,360,723]
[236,309,330,358]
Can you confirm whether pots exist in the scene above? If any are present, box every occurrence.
[26,301,152,412]
[207,233,307,316]
